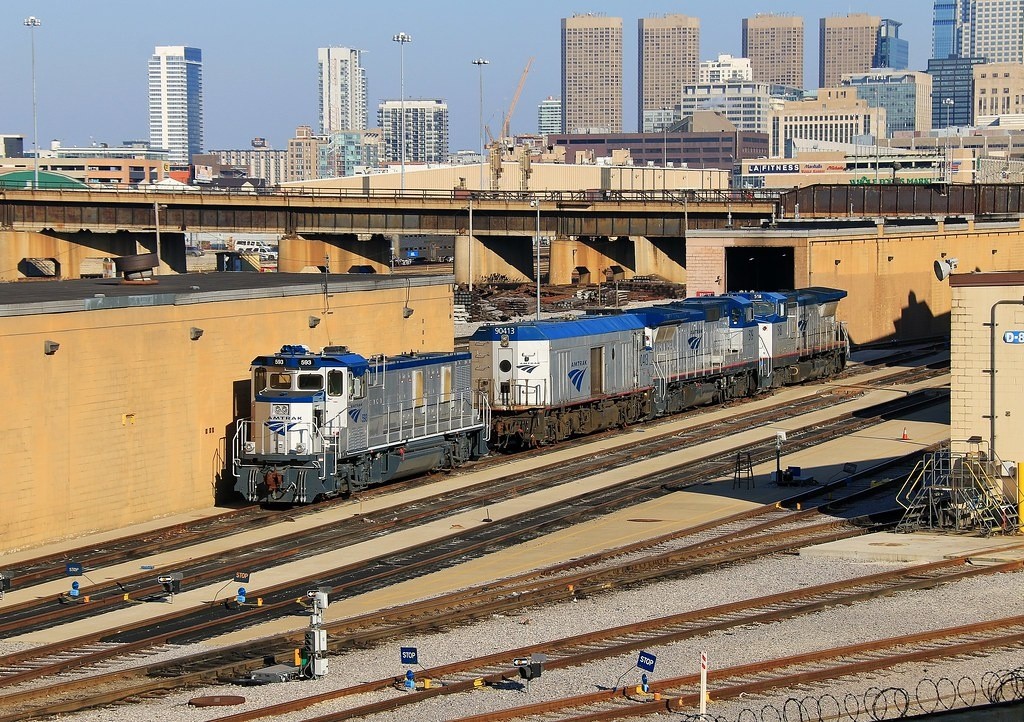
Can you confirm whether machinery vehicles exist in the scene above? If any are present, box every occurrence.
[484,57,535,163]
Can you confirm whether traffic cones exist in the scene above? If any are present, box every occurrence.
[901,427,909,440]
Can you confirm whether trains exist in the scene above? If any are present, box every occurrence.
[232,287,850,503]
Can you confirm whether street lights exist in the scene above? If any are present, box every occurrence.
[875,89,879,184]
[472,57,489,190]
[659,106,671,168]
[393,32,411,197]
[943,98,954,136]
[24,16,41,190]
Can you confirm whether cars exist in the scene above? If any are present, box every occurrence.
[185,240,278,261]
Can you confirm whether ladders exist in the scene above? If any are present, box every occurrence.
[732,452,755,490]
